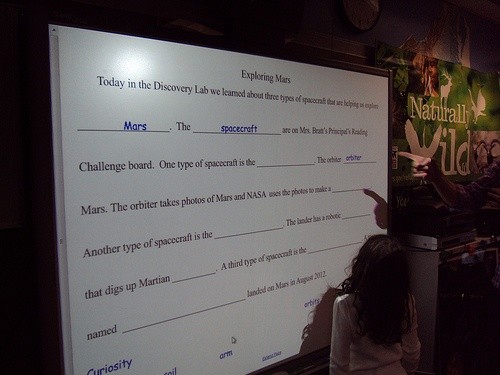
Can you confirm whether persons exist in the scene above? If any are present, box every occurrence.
[326,234,422,375]
[424,57,439,97]
[398,147,500,236]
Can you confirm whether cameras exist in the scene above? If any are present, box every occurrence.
[477,180,500,236]
